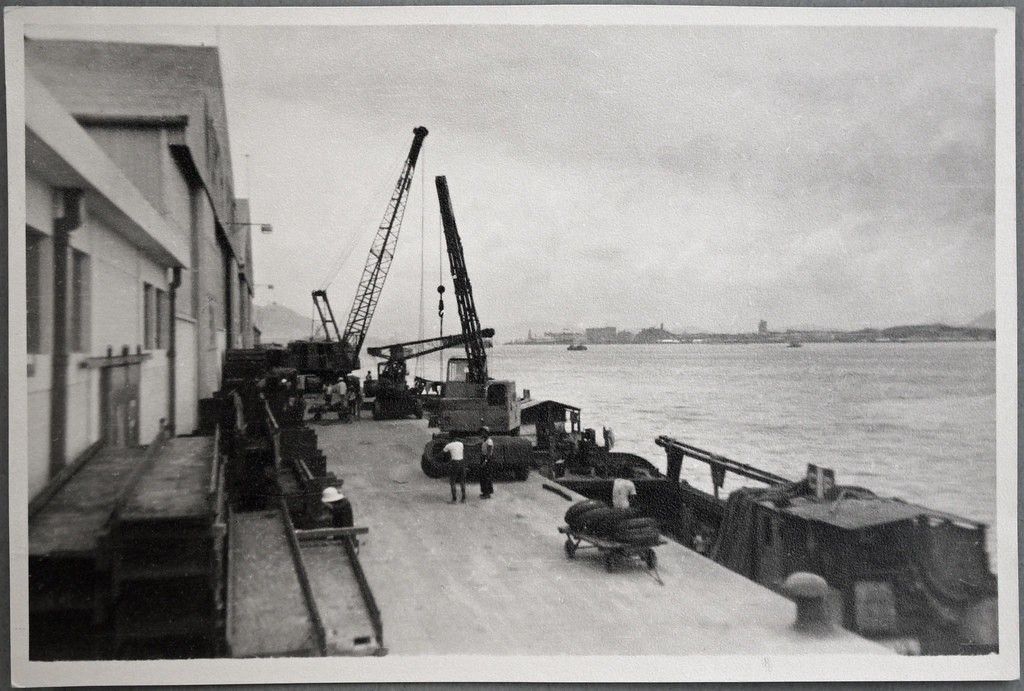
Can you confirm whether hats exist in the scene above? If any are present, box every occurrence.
[321,487,345,503]
[337,377,344,382]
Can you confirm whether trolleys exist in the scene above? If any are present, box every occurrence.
[557,521,668,572]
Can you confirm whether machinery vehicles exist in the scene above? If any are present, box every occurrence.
[284,127,496,423]
[417,174,535,482]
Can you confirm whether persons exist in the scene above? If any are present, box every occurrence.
[428,431,466,503]
[322,487,355,543]
[366,371,372,381]
[322,377,356,416]
[612,467,637,508]
[421,381,443,395]
[480,426,493,499]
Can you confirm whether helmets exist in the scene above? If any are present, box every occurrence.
[480,425,491,434]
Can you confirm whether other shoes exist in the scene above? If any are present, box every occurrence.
[480,493,491,500]
[488,490,493,494]
[459,499,466,503]
[446,500,457,505]
